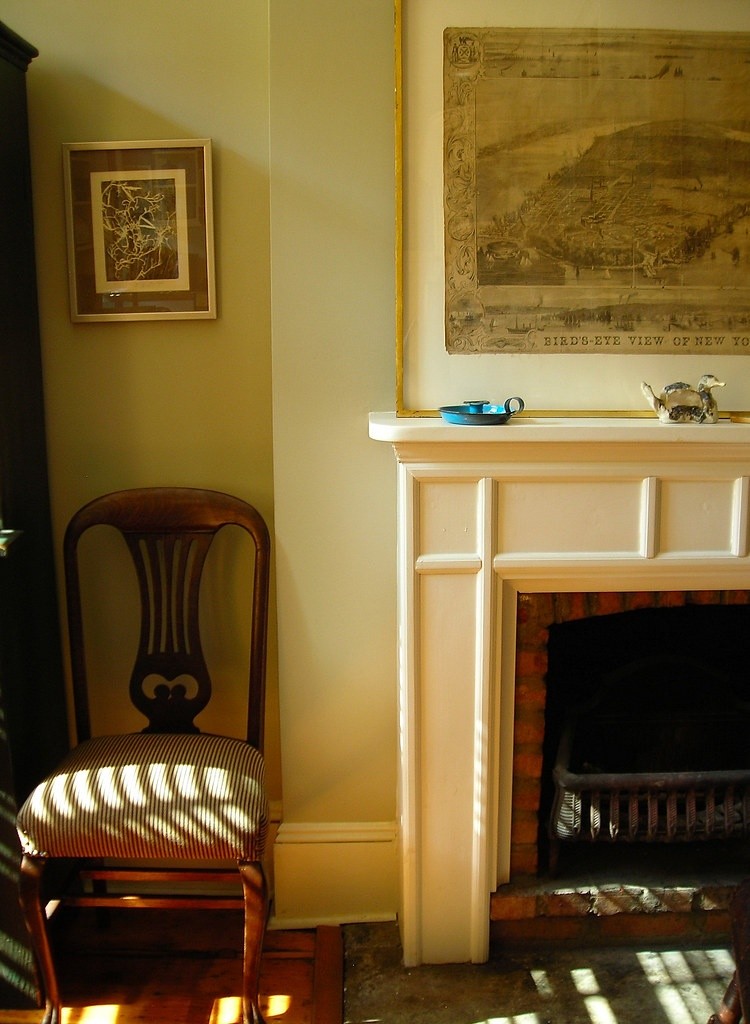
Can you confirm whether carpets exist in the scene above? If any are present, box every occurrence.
[339,921,750,1024]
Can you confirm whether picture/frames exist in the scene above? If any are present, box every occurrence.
[62,139,217,331]
[393,0,750,420]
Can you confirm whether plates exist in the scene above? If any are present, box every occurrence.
[439,405,515,424]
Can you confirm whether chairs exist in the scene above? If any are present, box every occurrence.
[14,488,271,1024]
